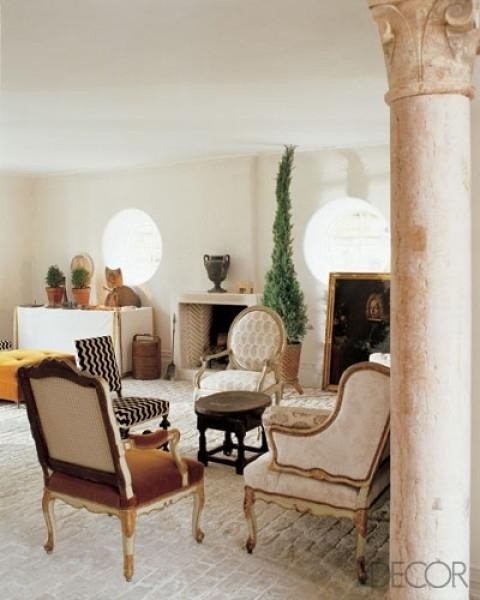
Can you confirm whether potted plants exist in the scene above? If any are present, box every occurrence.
[259,142,308,399]
[43,265,93,307]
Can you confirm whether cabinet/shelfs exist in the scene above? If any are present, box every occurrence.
[13,304,152,378]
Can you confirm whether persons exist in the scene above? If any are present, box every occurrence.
[366,293,389,320]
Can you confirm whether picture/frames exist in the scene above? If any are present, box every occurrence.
[322,270,393,393]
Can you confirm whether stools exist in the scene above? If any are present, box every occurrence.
[0,348,78,409]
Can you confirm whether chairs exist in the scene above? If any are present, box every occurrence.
[192,303,287,443]
[73,337,172,451]
[240,350,394,586]
[15,357,208,584]
